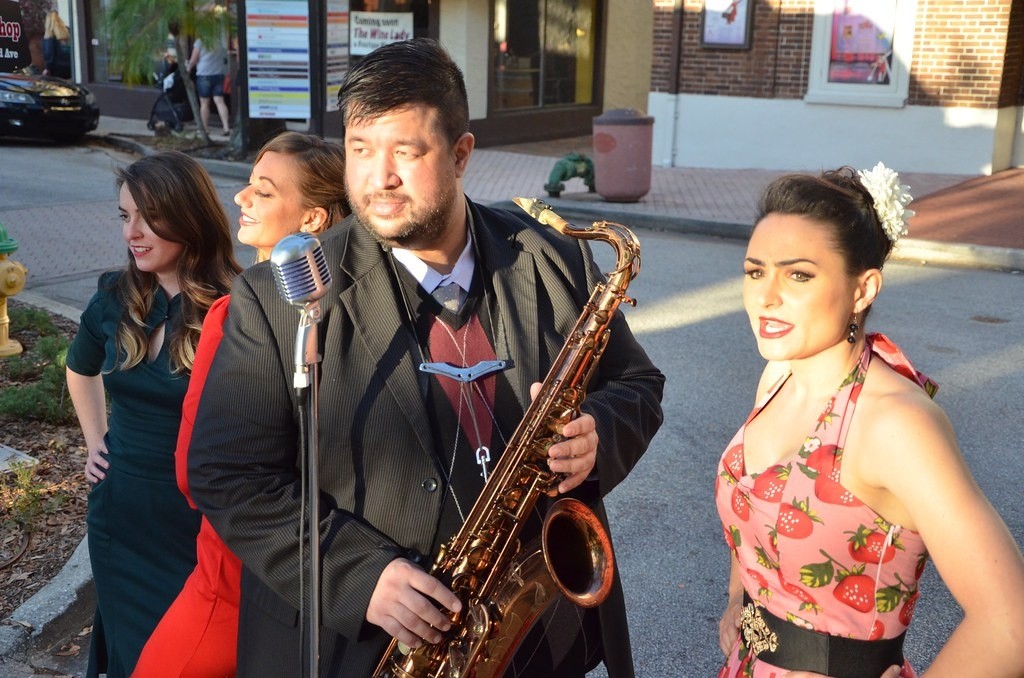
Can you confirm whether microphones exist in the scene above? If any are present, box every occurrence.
[269,231,330,406]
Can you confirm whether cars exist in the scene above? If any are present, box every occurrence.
[0,71,101,146]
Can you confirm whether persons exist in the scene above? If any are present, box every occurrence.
[131,132,353,678]
[716,161,1024,678]
[38,12,231,140]
[66,150,246,678]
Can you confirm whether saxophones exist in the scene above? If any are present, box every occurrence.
[370,194,639,678]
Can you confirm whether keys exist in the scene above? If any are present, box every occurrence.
[187,36,665,678]
[475,446,492,486]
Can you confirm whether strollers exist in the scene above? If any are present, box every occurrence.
[146,62,199,132]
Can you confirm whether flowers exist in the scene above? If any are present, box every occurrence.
[857,161,916,242]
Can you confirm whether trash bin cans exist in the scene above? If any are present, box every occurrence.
[592,107,656,204]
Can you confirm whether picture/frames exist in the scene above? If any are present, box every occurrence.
[699,0,755,51]
[804,0,916,108]
